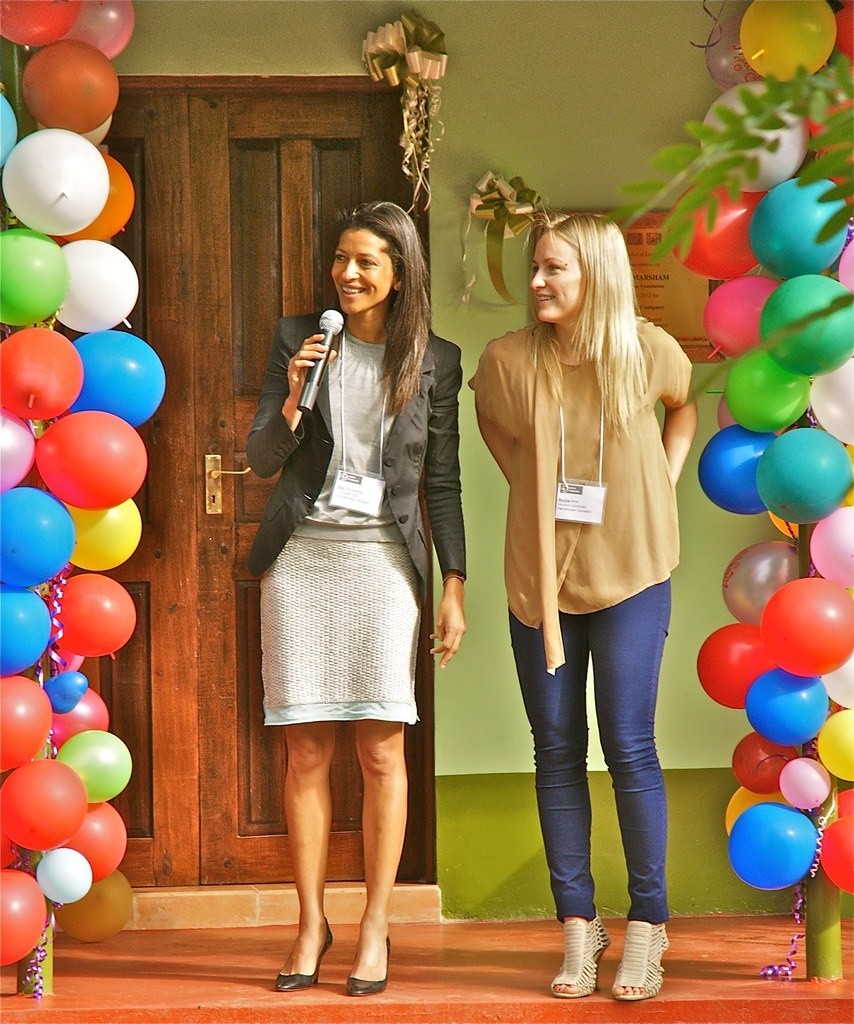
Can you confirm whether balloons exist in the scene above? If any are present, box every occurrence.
[667,0,854,895]
[0,0,167,968]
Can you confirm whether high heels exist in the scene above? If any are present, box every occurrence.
[611,920,670,1001]
[345,936,390,997]
[550,913,611,999]
[272,916,333,992]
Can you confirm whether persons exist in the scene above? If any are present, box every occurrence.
[470,210,699,1002]
[243,201,468,996]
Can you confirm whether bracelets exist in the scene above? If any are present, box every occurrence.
[443,574,464,585]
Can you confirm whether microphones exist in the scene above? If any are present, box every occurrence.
[297,309,345,414]
